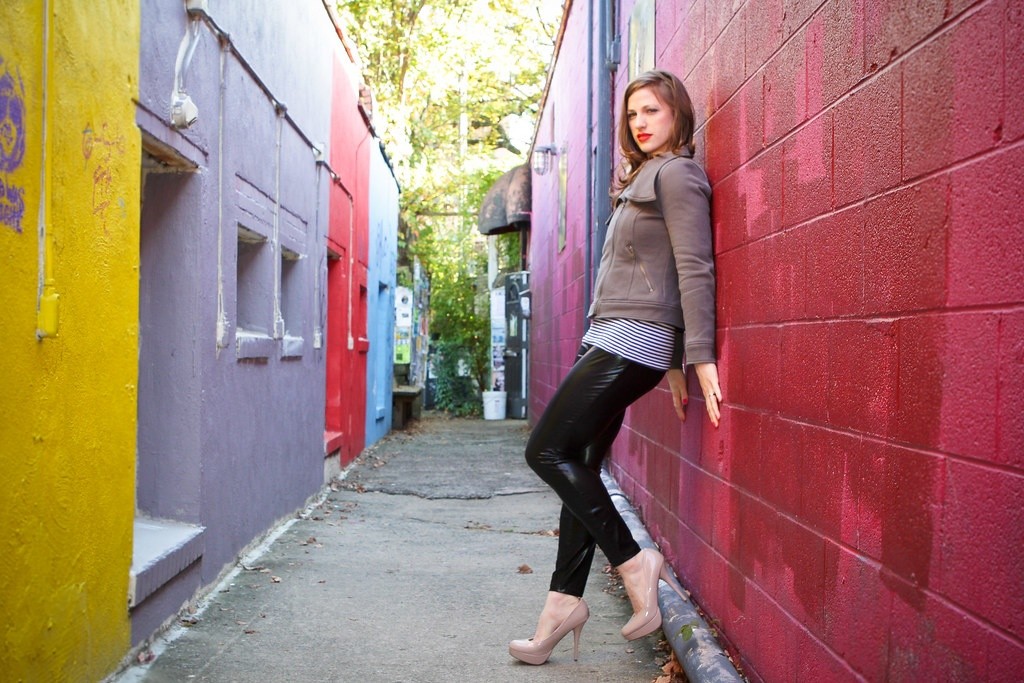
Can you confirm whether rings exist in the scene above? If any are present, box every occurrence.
[709,393,716,396]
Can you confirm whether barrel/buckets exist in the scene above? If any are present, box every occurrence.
[482,388,507,420]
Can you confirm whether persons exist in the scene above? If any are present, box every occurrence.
[509,67,721,667]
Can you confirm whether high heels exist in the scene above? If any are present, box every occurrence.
[509,597,589,665]
[620,547,688,642]
